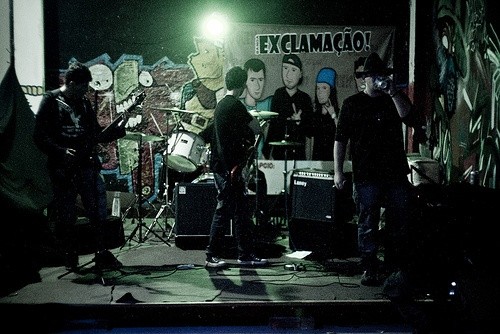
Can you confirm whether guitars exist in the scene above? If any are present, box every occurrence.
[62,91,148,191]
[230,119,270,194]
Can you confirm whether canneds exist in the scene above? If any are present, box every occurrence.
[376,78,387,89]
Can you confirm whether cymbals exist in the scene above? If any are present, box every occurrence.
[122,132,164,141]
[268,141,302,146]
[247,110,279,117]
[146,107,196,114]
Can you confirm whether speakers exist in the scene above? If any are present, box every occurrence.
[239,226,277,259]
[174,183,236,249]
[289,168,357,225]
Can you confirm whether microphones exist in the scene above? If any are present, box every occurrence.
[94,81,101,93]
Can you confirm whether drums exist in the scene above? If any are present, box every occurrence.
[205,146,211,168]
[163,129,206,172]
[191,172,215,183]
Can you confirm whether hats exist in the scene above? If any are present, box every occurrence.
[364,52,386,74]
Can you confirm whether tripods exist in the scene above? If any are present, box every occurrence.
[57,110,175,286]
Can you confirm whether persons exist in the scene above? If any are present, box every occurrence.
[33,63,132,269]
[333,52,419,285]
[206,67,267,267]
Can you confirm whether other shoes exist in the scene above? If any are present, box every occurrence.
[361,269,380,286]
[237,253,268,265]
[99,251,123,268]
[206,252,226,267]
